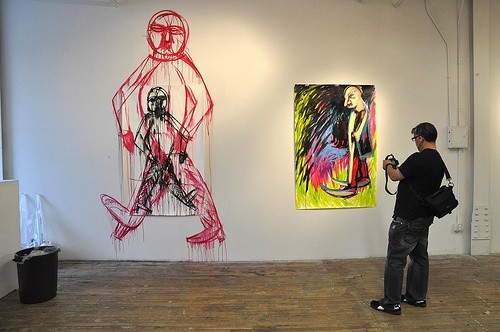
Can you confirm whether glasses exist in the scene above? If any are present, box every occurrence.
[412,135,420,141]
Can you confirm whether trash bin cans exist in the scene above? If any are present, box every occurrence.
[12,245,61,304]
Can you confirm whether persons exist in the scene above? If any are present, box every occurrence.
[370,122,459,316]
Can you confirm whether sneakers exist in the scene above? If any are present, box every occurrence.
[402,294,426,307]
[371,300,401,315]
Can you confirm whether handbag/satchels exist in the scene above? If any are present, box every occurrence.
[426,183,458,219]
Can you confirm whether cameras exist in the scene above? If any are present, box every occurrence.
[389,158,399,170]
